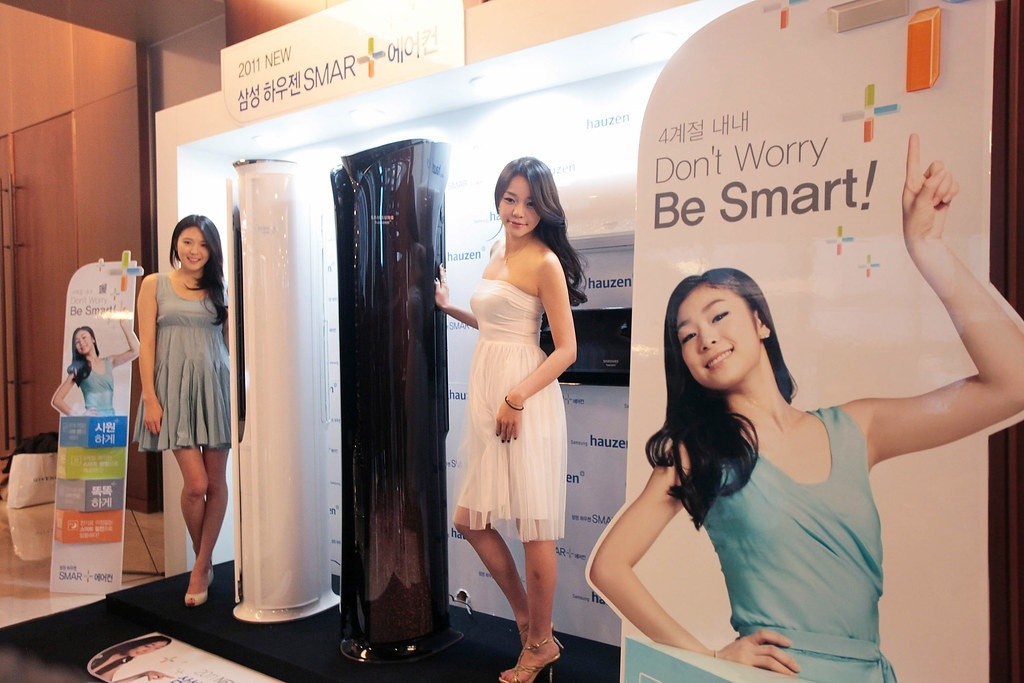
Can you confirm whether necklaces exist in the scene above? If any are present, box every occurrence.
[504,257,510,265]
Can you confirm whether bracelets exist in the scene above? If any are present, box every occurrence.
[505,396,524,411]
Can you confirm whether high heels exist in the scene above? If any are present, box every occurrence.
[499,638,561,683]
[519,620,564,650]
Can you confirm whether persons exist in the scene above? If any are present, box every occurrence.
[91,636,175,682]
[53,308,140,419]
[590,133,1024,683]
[131,215,231,606]
[434,158,586,682]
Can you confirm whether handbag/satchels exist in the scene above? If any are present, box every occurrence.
[7,453,57,509]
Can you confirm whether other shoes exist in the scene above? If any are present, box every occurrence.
[185,568,215,607]
